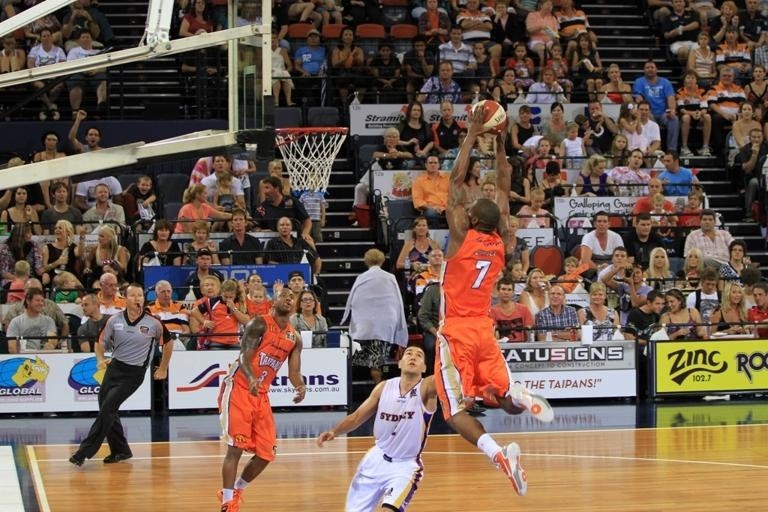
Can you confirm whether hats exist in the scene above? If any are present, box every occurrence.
[305,29,321,39]
[198,248,209,255]
[288,270,303,280]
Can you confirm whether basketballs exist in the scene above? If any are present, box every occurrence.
[470,99,508,138]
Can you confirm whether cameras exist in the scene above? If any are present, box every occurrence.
[624,267,634,278]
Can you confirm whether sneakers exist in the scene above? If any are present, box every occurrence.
[508,384,554,424]
[496,443,528,497]
[221,500,238,512]
[216,487,245,512]
[702,146,711,155]
[681,147,693,157]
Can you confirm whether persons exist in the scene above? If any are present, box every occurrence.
[433,102,555,498]
[317,345,444,512]
[214,287,307,512]
[69,284,175,469]
[1,0,768,384]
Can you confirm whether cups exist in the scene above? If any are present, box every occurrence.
[60,341,68,352]
[19,339,26,353]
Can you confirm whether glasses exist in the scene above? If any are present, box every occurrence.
[102,259,116,266]
[301,297,315,302]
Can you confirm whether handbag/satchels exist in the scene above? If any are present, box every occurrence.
[198,330,207,345]
[396,344,405,361]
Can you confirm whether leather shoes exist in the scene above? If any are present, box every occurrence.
[69,453,85,467]
[103,450,133,464]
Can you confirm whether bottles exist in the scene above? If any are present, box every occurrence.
[546,331,553,341]
[529,333,535,342]
[405,256,410,268]
